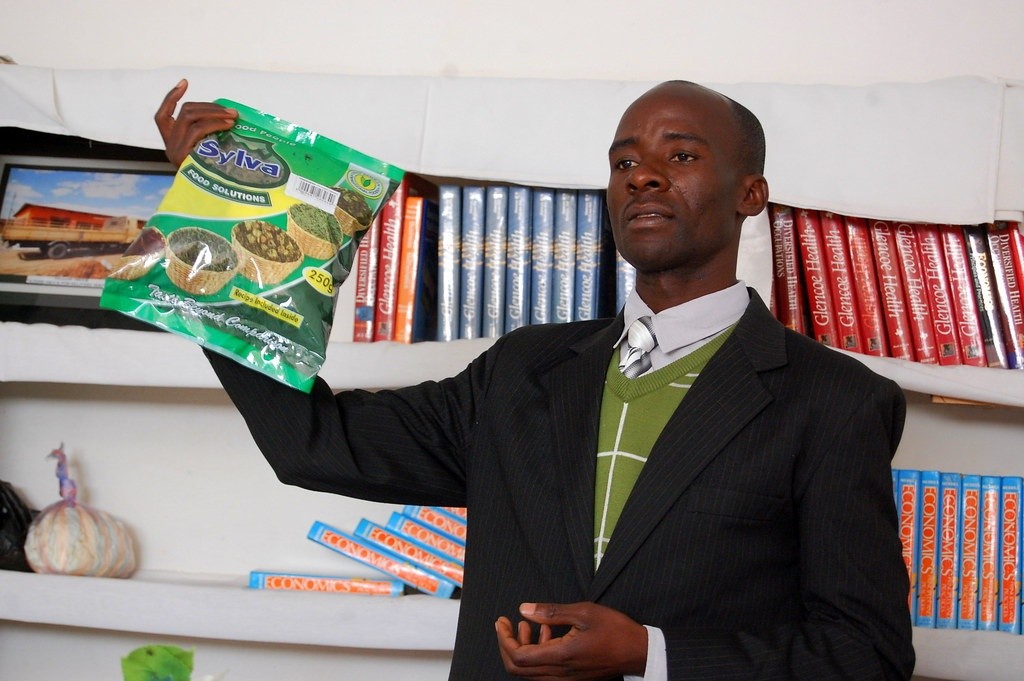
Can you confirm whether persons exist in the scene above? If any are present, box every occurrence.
[153,78,915,681]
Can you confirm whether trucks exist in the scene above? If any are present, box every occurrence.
[2,216,151,261]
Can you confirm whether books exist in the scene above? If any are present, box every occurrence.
[766,201,1024,370]
[248,505,467,599]
[890,468,1024,635]
[352,172,637,342]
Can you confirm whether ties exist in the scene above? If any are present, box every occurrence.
[619,315,662,380]
